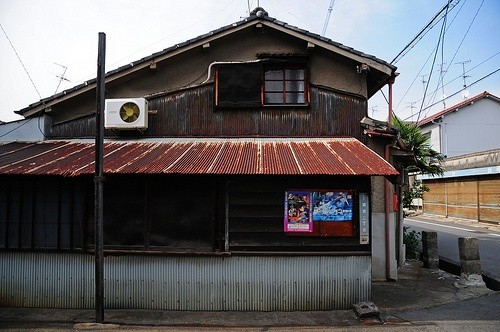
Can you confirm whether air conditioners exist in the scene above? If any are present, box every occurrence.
[104,98,149,129]
